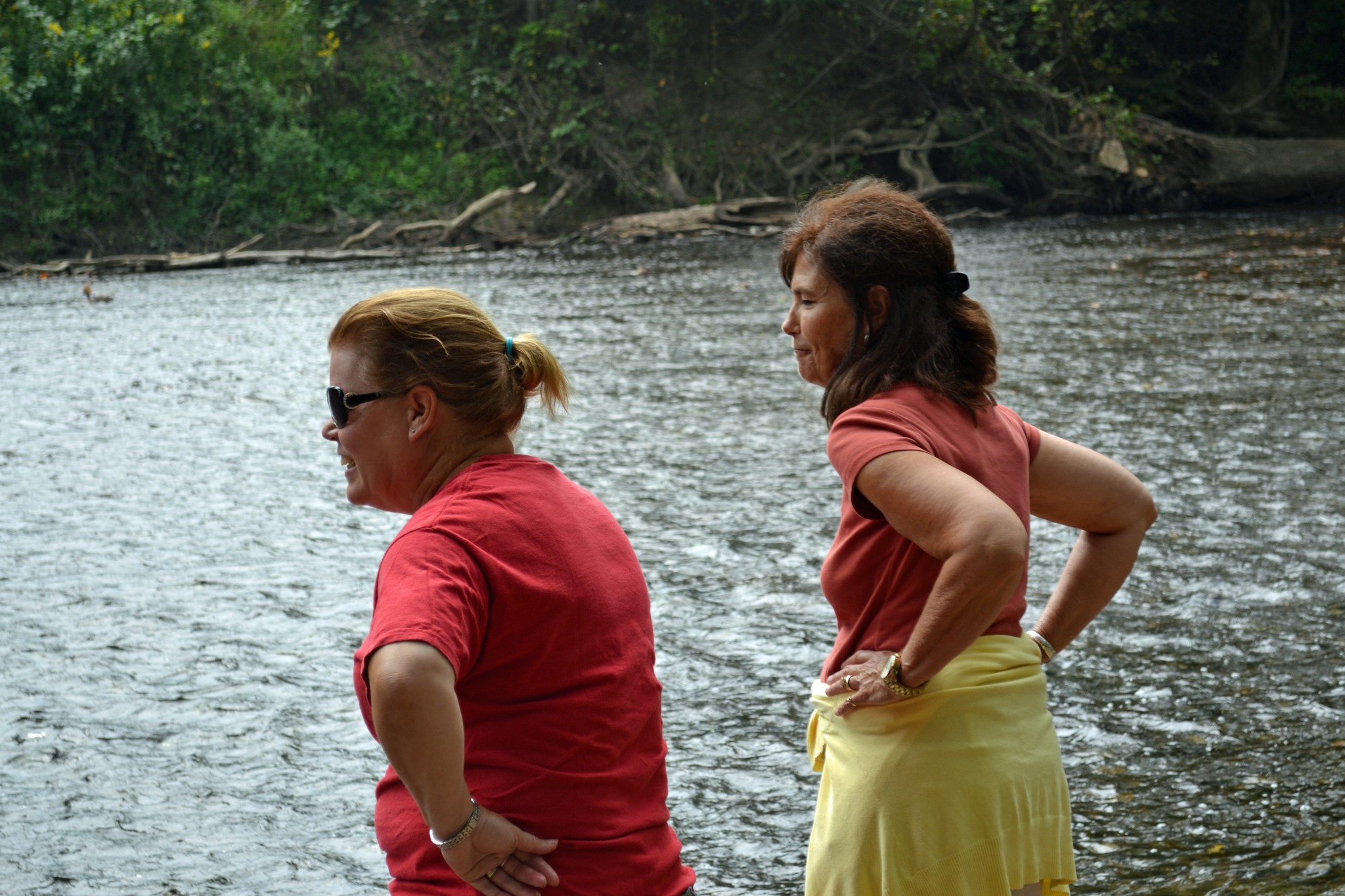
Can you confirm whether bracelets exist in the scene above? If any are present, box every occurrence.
[429,797,481,850]
[1025,631,1056,663]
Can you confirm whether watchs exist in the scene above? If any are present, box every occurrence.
[881,647,932,698]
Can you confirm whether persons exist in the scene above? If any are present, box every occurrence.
[322,288,698,896]
[780,179,1158,896]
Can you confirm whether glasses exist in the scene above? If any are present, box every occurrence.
[326,385,402,428]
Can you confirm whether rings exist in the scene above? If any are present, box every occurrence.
[500,854,511,866]
[841,675,855,691]
[848,695,858,707]
[487,867,501,879]
